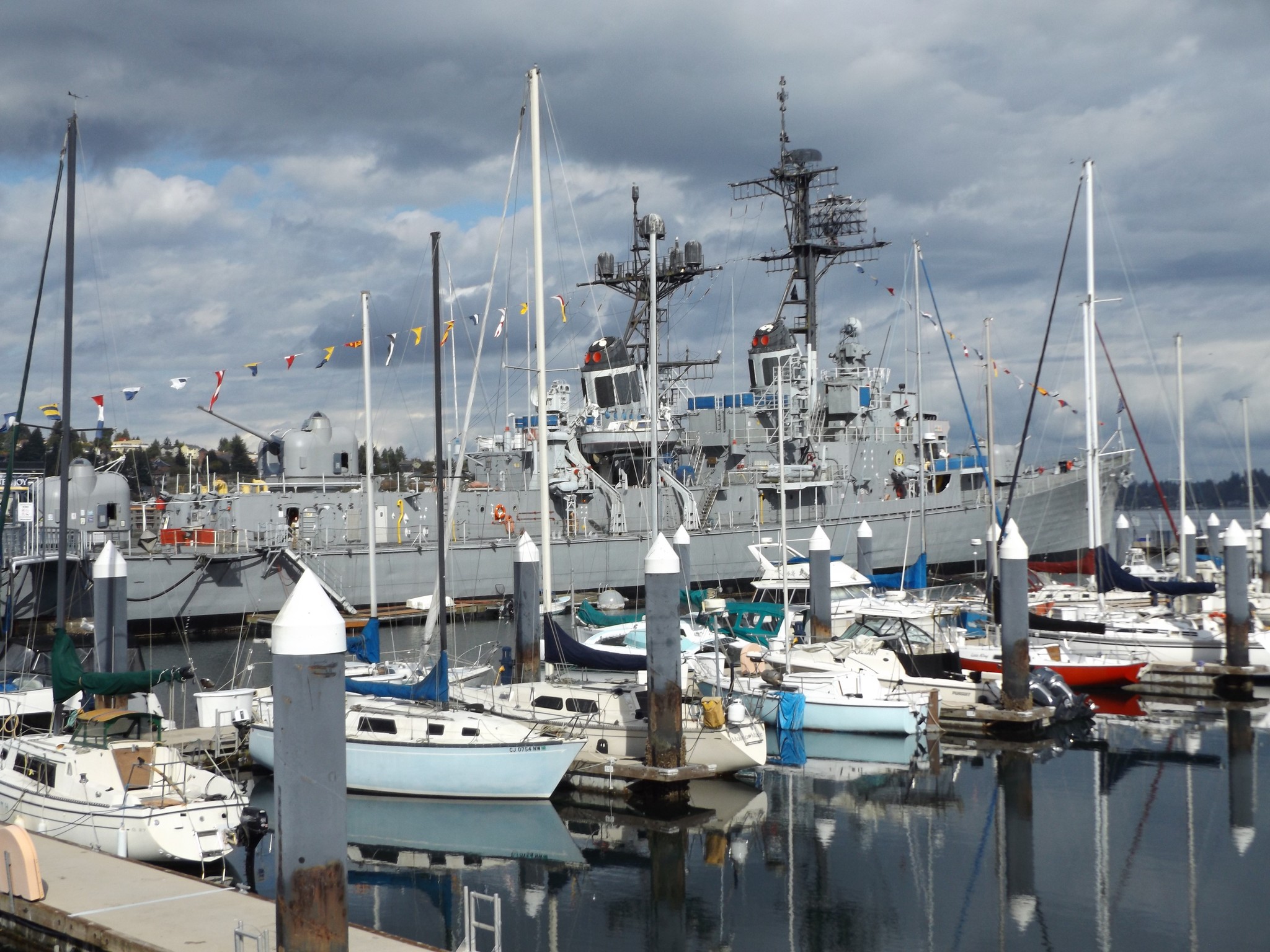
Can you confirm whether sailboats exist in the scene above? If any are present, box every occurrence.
[194,59,1270,801]
[234,774,941,952]
[0,94,248,871]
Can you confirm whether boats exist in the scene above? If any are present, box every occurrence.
[0,69,1119,621]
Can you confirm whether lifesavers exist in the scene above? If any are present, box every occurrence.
[896,485,904,497]
[1210,611,1227,619]
[1039,466,1044,473]
[895,422,901,434]
[1066,460,1073,470]
[495,504,506,519]
